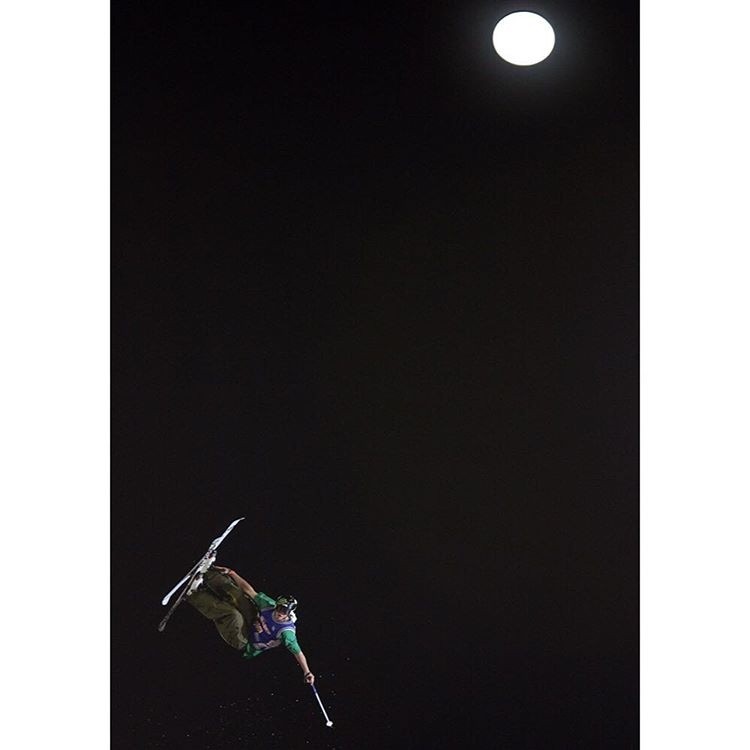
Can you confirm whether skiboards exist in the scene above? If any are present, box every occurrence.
[157,517,246,632]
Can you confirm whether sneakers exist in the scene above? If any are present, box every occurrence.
[199,550,217,573]
[182,573,205,601]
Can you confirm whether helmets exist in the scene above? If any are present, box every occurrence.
[275,594,298,617]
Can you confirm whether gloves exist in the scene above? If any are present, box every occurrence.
[210,564,232,576]
[303,672,315,687]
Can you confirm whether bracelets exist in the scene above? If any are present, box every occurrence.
[303,672,311,680]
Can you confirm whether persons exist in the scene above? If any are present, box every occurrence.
[185,557,314,687]
